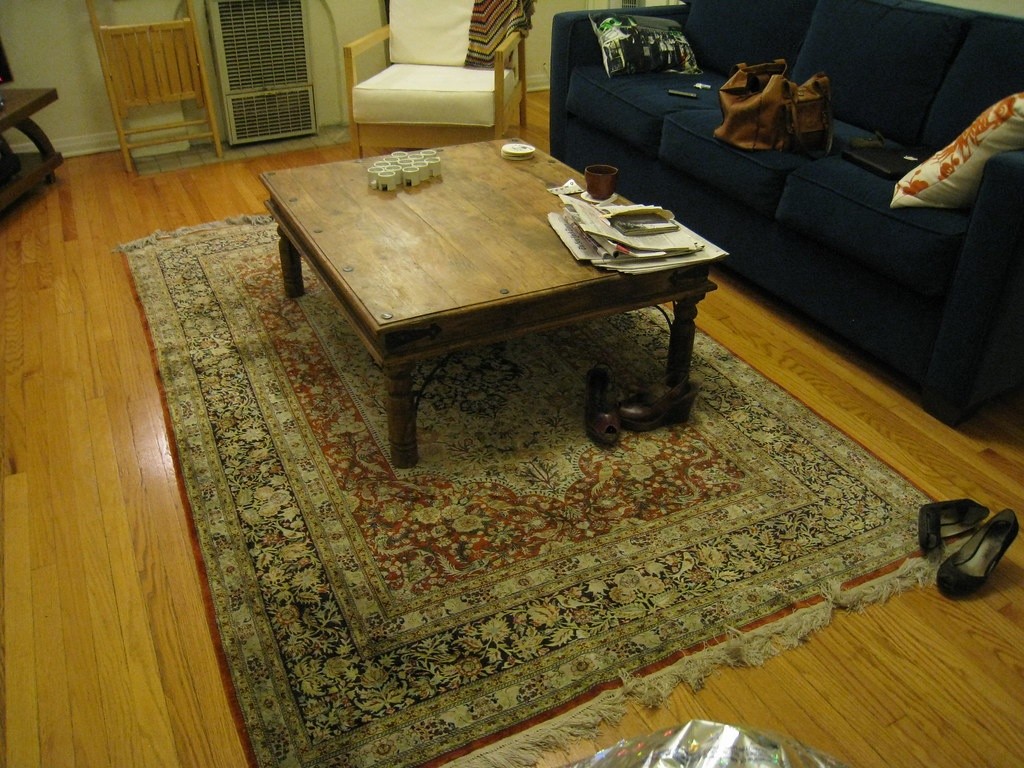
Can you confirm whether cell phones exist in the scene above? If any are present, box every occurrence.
[668,89,696,99]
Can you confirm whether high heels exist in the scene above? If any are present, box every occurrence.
[937,508,1019,597]
[584,362,620,446]
[620,366,698,432]
[919,498,990,551]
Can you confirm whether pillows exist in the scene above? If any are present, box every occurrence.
[588,8,704,79]
[890,93,1024,208]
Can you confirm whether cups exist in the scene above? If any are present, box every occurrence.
[583,164,619,200]
[368,149,441,192]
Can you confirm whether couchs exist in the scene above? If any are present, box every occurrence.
[551,0,1024,431]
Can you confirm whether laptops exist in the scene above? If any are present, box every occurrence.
[841,146,930,180]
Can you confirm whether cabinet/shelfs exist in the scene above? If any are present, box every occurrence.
[0,87,65,213]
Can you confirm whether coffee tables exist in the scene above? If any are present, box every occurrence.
[258,138,719,470]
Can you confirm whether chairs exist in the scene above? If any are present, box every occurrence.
[343,1,539,163]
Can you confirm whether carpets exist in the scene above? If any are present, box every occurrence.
[109,214,978,768]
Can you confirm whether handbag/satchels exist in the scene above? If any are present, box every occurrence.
[712,59,835,159]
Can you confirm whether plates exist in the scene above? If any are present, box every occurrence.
[580,190,618,203]
